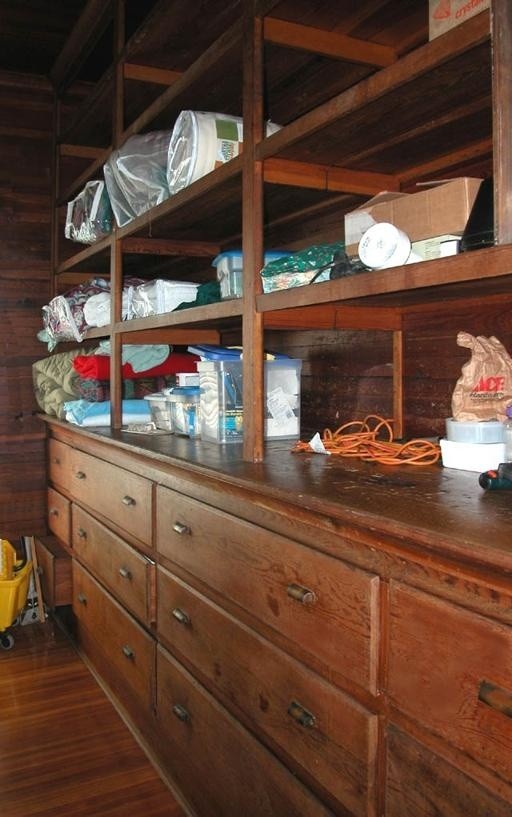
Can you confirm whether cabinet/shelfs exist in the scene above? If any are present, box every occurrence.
[256,15,501,465]
[49,5,112,427]
[116,16,253,451]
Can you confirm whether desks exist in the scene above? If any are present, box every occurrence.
[33,412,511,817]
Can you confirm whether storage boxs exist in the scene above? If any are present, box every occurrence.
[211,249,292,300]
[343,177,484,257]
[186,346,302,447]
[428,0,490,41]
[144,393,171,430]
[168,387,201,441]
